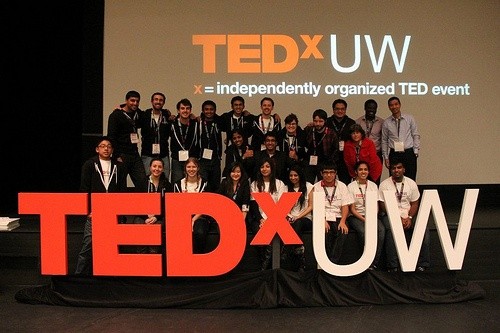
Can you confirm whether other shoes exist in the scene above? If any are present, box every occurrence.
[387,268,398,273]
[417,266,424,272]
[366,264,377,272]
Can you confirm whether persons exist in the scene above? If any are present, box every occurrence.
[74,90,429,284]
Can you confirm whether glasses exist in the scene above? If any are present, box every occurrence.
[322,171,337,176]
